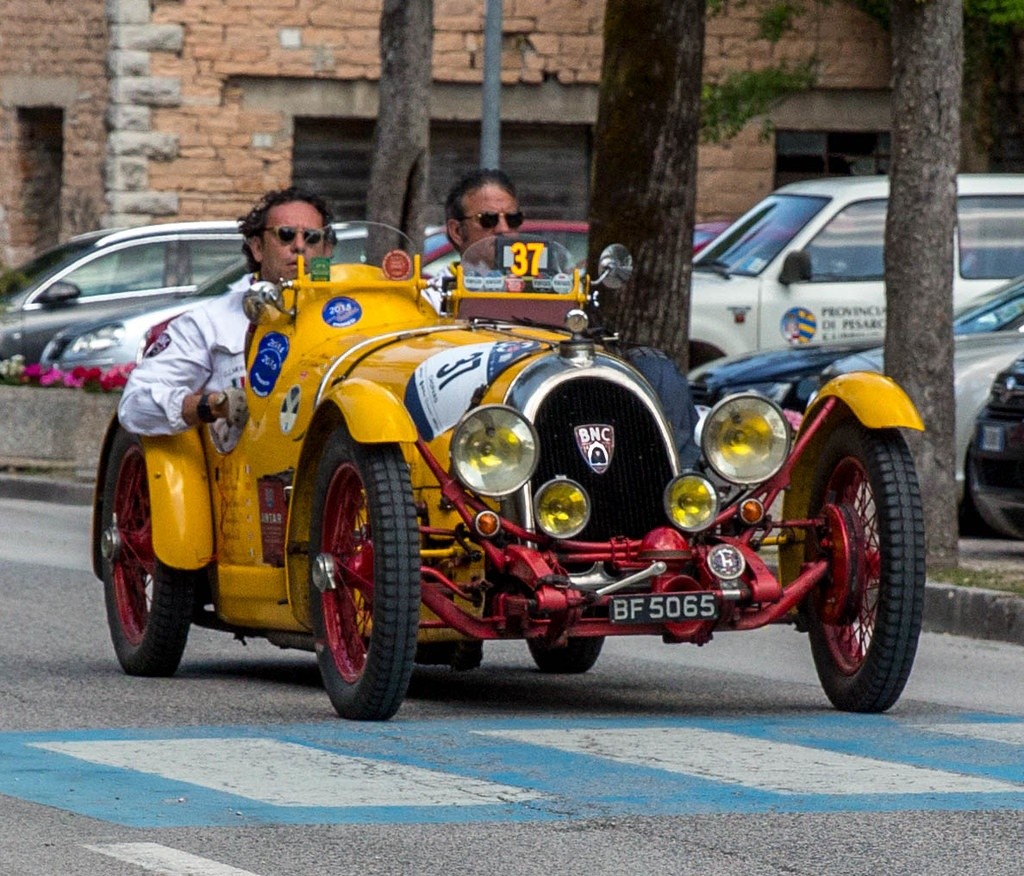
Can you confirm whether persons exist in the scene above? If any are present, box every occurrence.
[118,186,339,453]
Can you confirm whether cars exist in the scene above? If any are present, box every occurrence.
[39,227,368,377]
[806,328,1023,540]
[0,221,351,367]
[692,218,799,252]
[93,221,927,724]
[418,220,591,280]
[688,270,1023,415]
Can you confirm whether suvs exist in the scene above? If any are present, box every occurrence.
[687,173,1024,369]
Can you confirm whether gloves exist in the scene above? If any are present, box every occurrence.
[216,388,249,427]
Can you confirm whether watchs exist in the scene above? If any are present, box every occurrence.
[416,166,526,315]
[198,392,220,424]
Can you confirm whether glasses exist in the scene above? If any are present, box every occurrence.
[464,210,525,229]
[264,225,326,245]
